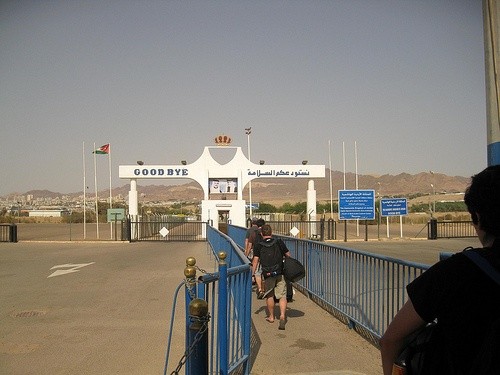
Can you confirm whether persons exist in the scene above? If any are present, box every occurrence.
[245,217,266,299]
[380,164,500,375]
[252,223,290,330]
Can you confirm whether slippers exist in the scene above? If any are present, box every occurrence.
[279,319,286,330]
[266,318,274,323]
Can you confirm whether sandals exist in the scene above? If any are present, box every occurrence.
[258,290,264,299]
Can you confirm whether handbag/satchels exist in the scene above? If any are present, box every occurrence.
[391,322,445,375]
[284,257,305,283]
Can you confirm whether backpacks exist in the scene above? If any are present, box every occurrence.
[254,231,263,256]
[260,238,283,276]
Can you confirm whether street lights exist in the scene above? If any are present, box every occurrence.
[244,127,252,220]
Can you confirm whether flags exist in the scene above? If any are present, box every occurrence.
[92,144,109,154]
